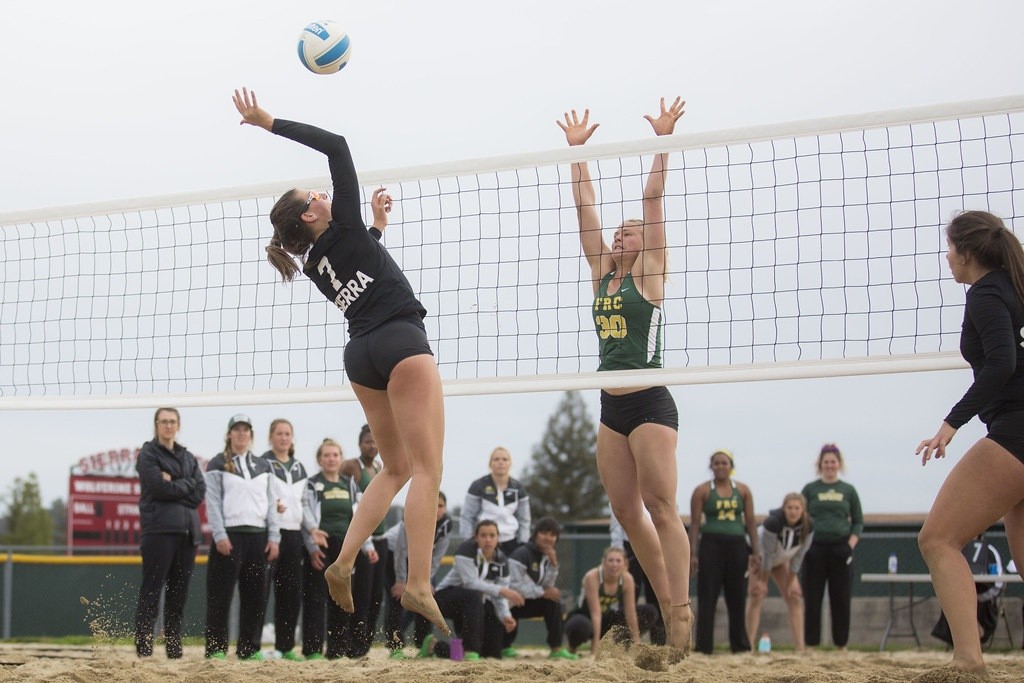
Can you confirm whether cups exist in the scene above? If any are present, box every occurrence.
[451,639,463,661]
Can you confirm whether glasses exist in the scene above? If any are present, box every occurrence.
[299,191,319,217]
[157,418,177,426]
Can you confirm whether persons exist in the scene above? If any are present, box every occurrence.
[688,450,760,655]
[745,493,813,653]
[556,96,695,666]
[136,407,207,658]
[201,415,576,660]
[608,497,660,615]
[231,88,452,636]
[800,444,865,650]
[563,546,640,659]
[915,210,1024,681]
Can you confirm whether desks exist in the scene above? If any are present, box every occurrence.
[861,573,1024,652]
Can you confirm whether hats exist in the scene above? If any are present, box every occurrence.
[228,414,252,431]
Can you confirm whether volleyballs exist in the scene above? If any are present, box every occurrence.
[297,19,353,75]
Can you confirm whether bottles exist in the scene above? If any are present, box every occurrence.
[758,632,771,653]
[888,552,898,573]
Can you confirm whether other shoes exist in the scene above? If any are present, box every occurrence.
[282,651,301,662]
[463,651,479,660]
[549,649,578,660]
[208,650,226,660]
[242,652,262,660]
[390,650,405,659]
[415,634,435,658]
[305,653,325,662]
[503,648,516,657]
